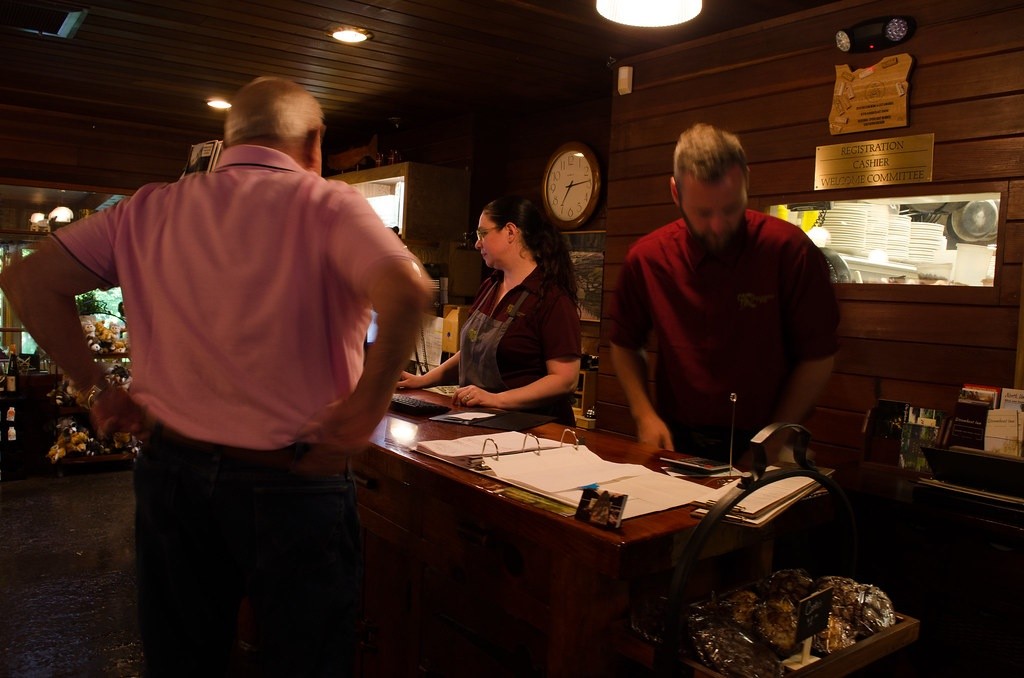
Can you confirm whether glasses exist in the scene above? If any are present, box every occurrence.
[475,222,507,241]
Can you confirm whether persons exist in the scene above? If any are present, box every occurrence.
[0,74,436,678]
[607,125,844,461]
[396,197,582,427]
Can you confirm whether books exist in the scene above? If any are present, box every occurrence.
[873,382,1024,476]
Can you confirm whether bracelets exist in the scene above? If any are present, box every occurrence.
[82,377,109,410]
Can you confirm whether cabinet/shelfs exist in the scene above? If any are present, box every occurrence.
[54,351,136,478]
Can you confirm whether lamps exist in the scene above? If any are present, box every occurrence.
[28,188,75,231]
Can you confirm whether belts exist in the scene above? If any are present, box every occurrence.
[151,419,349,476]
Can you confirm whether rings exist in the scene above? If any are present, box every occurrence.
[466,395,470,400]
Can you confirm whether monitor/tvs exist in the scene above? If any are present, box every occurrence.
[365,309,380,345]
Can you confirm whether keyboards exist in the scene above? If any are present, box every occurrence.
[389,394,452,417]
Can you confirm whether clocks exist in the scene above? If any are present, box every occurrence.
[541,141,601,231]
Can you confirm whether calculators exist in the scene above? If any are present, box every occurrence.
[659,457,732,475]
